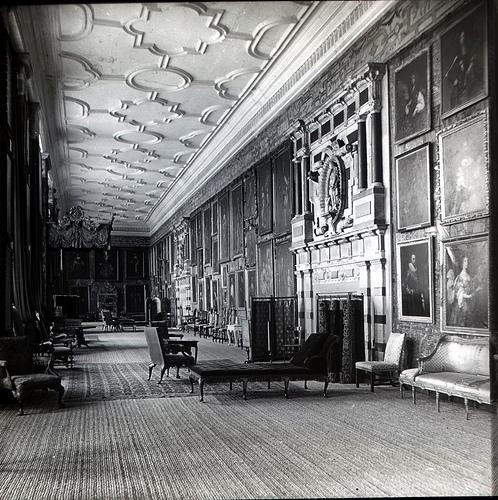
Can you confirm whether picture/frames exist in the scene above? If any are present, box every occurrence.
[381,3,490,335]
[188,135,295,313]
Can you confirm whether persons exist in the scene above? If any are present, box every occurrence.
[446,255,488,328]
[442,31,485,107]
[403,252,427,316]
[397,72,431,141]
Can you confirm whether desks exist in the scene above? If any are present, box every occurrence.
[226,323,242,348]
[68,325,97,349]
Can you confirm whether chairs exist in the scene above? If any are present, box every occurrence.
[0,322,75,416]
[355,333,444,405]
[146,309,235,383]
[101,310,119,333]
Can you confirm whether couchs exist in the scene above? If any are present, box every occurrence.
[413,334,498,421]
[188,333,342,401]
